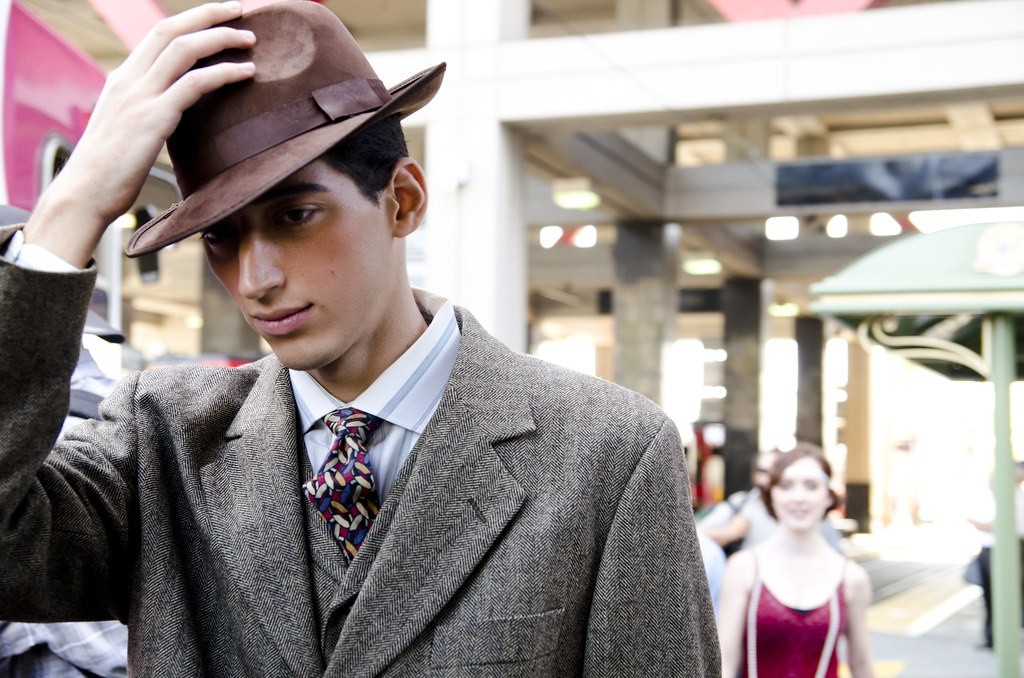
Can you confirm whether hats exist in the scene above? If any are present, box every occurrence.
[125,1,447,258]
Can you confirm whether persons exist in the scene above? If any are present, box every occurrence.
[2,1,724,678]
[965,459,1023,649]
[694,439,872,678]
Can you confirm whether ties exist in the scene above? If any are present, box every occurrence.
[301,408,385,564]
